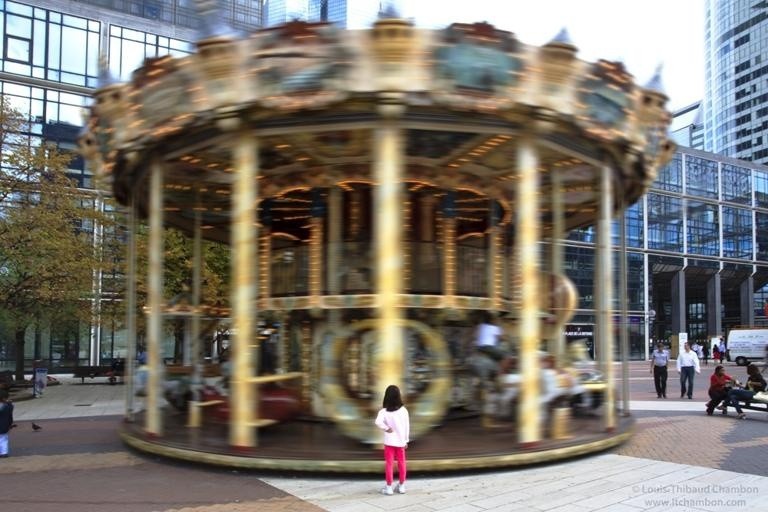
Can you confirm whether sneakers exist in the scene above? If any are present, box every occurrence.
[735,413,746,420]
[394,483,406,494]
[717,405,727,410]
[380,484,393,495]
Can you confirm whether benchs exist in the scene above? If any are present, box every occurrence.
[720,378,768,415]
[73,365,124,384]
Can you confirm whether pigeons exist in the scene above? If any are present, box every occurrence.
[32,422,42,432]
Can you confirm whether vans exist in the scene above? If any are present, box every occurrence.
[725,328,768,366]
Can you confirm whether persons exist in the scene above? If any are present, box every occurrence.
[704,365,737,415]
[691,337,726,366]
[676,341,700,399]
[649,343,669,399]
[375,384,410,496]
[0,389,13,459]
[136,351,148,365]
[475,316,505,362]
[715,363,767,419]
